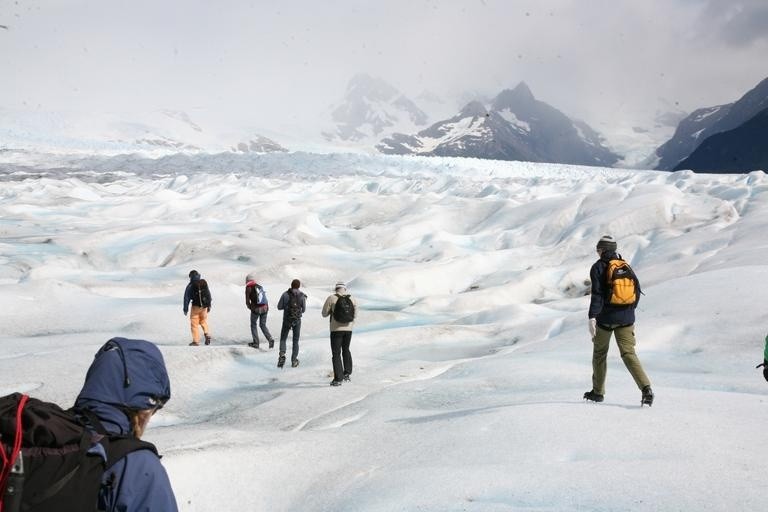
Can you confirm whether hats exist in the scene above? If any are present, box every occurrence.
[335,281,346,291]
[246,274,254,282]
[597,235,617,251]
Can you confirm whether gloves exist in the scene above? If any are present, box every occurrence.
[588,318,596,337]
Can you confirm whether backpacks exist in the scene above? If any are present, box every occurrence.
[250,285,267,306]
[287,289,304,320]
[0,391,163,512]
[596,254,645,308]
[329,293,354,323]
[191,279,210,307]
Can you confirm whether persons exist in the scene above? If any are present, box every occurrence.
[583,236,655,407]
[277,279,307,369]
[183,270,212,345]
[322,280,356,386]
[244,273,275,349]
[1,336,181,512]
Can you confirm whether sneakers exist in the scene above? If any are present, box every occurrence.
[248,343,258,348]
[277,355,285,368]
[584,391,603,402]
[292,359,298,367]
[330,379,342,386]
[189,342,200,346]
[269,339,274,348]
[204,336,210,345]
[640,388,653,407]
